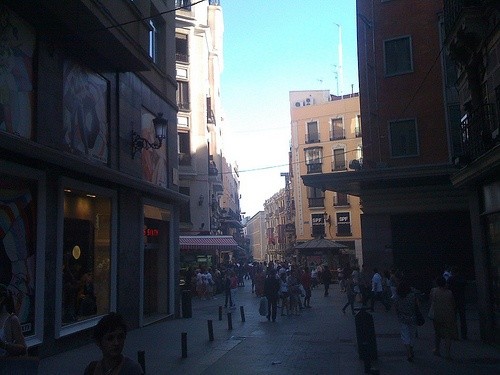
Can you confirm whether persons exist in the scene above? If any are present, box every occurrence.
[0,284,27,358]
[185,259,469,375]
[83,312,145,375]
[63,270,96,322]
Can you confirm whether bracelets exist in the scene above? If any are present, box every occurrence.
[4,341,8,349]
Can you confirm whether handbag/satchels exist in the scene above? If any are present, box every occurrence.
[429,288,437,316]
[258,296,268,315]
[0,358,41,375]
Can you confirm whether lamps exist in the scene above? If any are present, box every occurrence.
[131,112,168,153]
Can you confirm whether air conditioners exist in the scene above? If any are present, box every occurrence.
[304,98,314,106]
[293,101,303,108]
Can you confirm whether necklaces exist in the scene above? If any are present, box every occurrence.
[100,355,123,375]
